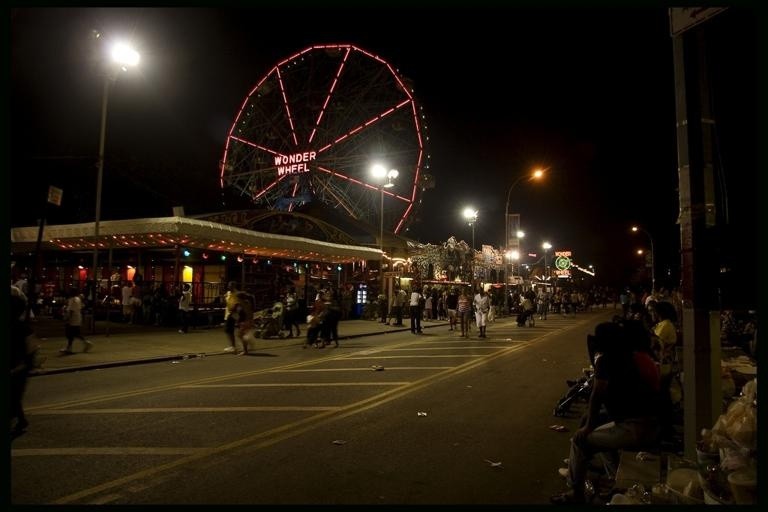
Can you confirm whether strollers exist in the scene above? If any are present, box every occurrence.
[254,302,286,339]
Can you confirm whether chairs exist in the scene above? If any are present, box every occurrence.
[613,360,683,485]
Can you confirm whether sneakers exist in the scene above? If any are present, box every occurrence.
[303,340,339,349]
[449,326,486,338]
[550,458,590,506]
[83,340,93,351]
[224,345,248,355]
[59,348,73,354]
[411,330,423,334]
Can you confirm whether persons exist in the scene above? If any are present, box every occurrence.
[4,286,36,437]
[378,282,549,337]
[214,282,378,354]
[9,279,86,319]
[86,275,191,333]
[549,286,758,509]
[59,287,94,352]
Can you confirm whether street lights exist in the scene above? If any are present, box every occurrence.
[362,153,400,251]
[89,24,156,314]
[503,165,546,316]
[630,224,657,299]
[459,203,479,251]
